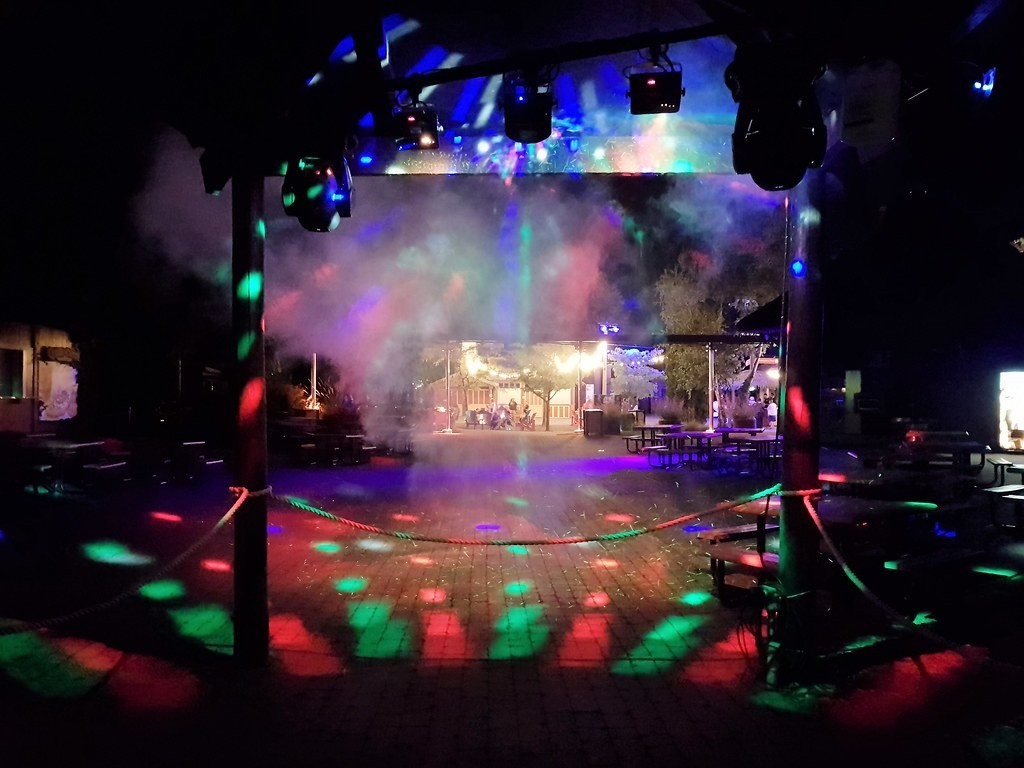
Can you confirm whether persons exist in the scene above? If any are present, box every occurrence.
[509,398,517,421]
[343,391,354,408]
[524,401,529,417]
[763,393,778,422]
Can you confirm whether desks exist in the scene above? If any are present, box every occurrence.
[713,426,765,444]
[732,494,872,581]
[737,434,783,463]
[908,441,990,474]
[913,428,976,441]
[632,423,683,449]
[660,432,722,466]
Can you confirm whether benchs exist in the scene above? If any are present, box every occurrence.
[622,406,1024,631]
